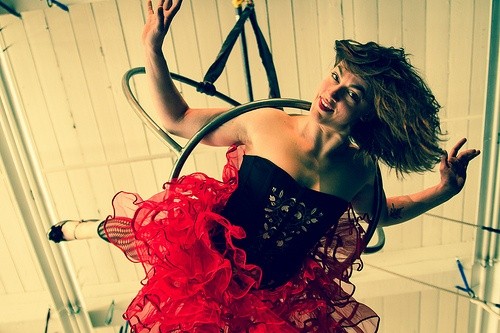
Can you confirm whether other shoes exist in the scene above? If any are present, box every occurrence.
[48,219,99,242]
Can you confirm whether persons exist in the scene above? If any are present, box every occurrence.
[45,0,482,333]
